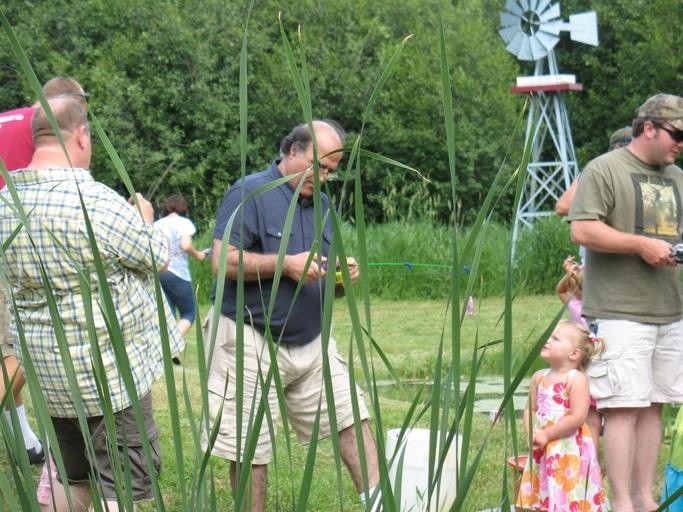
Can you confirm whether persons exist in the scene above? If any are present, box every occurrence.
[556,254,600,470]
[553,127,639,264]
[564,91,682,512]
[0,76,90,467]
[149,193,208,367]
[2,98,172,512]
[197,117,394,512]
[511,318,609,512]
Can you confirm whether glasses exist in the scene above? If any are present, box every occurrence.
[655,124,683,142]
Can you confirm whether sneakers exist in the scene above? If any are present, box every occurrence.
[27,440,46,467]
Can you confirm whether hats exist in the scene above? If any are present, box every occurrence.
[610,127,633,143]
[634,94,683,132]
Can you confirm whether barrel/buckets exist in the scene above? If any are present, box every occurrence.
[509,454,528,511]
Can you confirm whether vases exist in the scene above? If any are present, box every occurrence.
[505,451,527,508]
